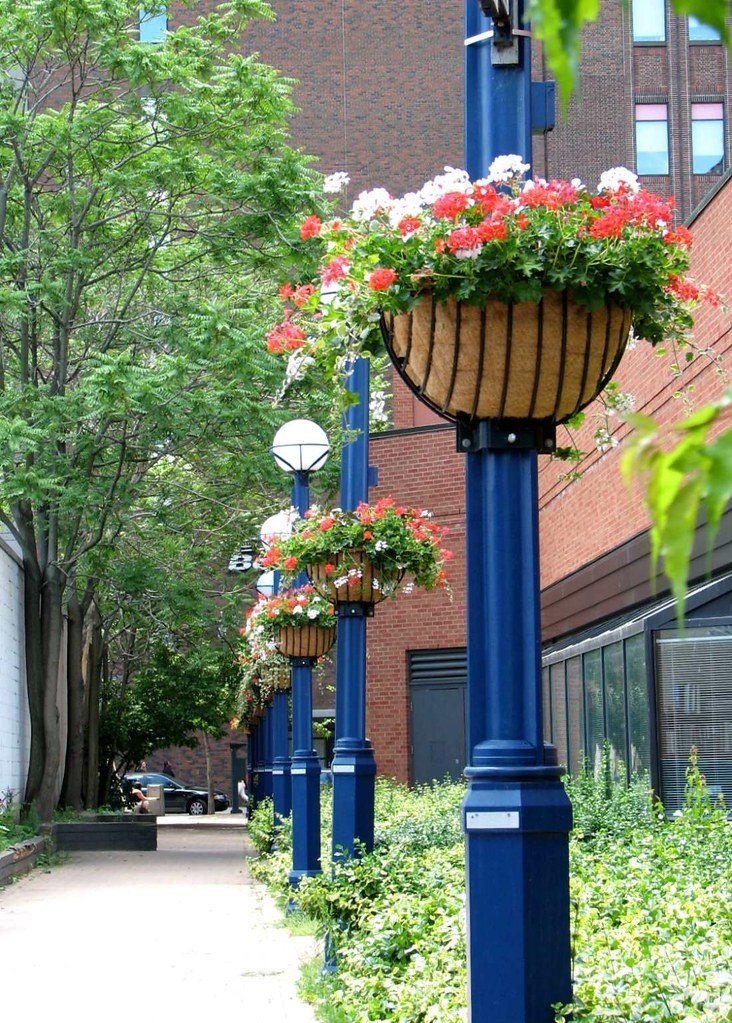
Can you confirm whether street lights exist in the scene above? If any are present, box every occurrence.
[255,419,335,918]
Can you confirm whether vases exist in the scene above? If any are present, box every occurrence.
[301,546,409,605]
[377,289,634,422]
[255,661,294,690]
[267,618,339,660]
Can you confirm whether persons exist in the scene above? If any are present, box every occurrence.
[137,762,146,772]
[131,782,160,813]
[163,761,175,777]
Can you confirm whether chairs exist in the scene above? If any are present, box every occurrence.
[144,783,167,816]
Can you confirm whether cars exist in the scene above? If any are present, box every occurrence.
[118,773,230,815]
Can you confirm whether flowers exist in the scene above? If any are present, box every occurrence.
[218,485,451,724]
[270,141,722,345]
